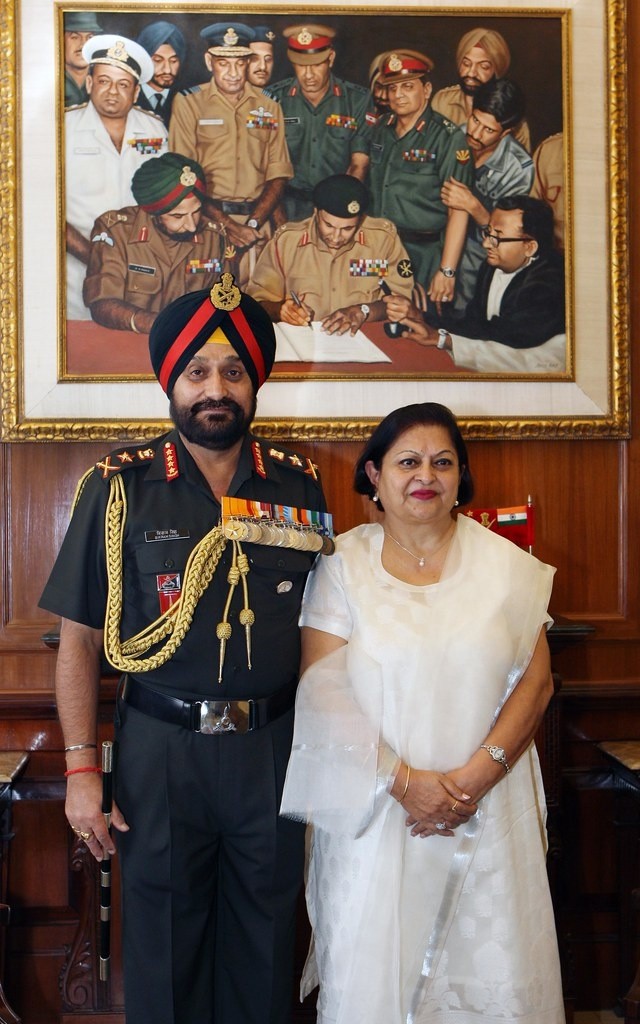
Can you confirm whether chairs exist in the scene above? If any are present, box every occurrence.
[4,799,110,1024]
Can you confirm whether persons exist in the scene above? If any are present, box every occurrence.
[280,402,566,1024]
[39,272,332,1024]
[63,12,571,375]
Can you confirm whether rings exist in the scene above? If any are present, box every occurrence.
[72,826,81,834]
[450,801,459,814]
[436,821,447,830]
[443,297,448,302]
[81,832,95,841]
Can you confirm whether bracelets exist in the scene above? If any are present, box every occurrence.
[64,766,102,777]
[397,765,411,803]
[130,308,143,334]
[64,744,98,751]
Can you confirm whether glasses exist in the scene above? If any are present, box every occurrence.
[481,229,533,248]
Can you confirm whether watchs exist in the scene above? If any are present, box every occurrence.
[436,328,448,350]
[356,303,370,322]
[439,267,456,277]
[480,744,511,773]
[245,218,261,231]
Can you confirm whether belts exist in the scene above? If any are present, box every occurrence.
[288,187,312,201]
[121,673,299,735]
[397,228,441,244]
[208,198,258,215]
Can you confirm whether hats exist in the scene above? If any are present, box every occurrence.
[81,35,154,84]
[368,52,386,93]
[313,175,369,218]
[379,50,433,85]
[148,274,276,397]
[283,24,336,65]
[249,26,276,44]
[137,21,186,63]
[63,12,103,34]
[131,152,206,216]
[200,23,256,57]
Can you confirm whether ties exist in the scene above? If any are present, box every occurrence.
[154,94,162,114]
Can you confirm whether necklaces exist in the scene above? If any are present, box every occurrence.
[385,531,452,567]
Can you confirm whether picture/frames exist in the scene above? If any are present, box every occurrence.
[0,0,628,444]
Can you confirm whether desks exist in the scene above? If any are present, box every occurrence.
[69,320,480,375]
[593,739,640,1017]
[0,750,31,1024]
